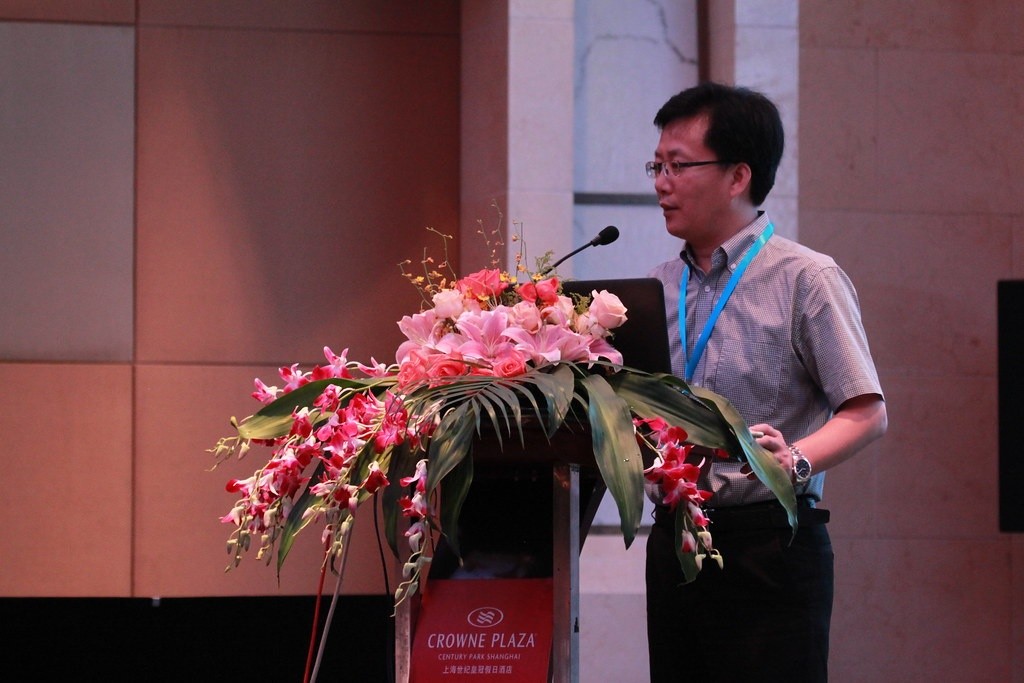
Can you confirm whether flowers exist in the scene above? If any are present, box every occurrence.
[205,197,799,618]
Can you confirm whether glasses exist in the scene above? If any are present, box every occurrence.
[646,160,743,176]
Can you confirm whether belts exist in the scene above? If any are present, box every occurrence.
[655,497,830,525]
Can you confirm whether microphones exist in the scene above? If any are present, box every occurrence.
[539,226,620,275]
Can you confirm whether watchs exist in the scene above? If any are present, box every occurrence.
[786,443,812,487]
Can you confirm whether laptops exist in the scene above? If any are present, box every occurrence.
[559,278,673,389]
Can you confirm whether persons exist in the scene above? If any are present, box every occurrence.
[645,80,889,683]
